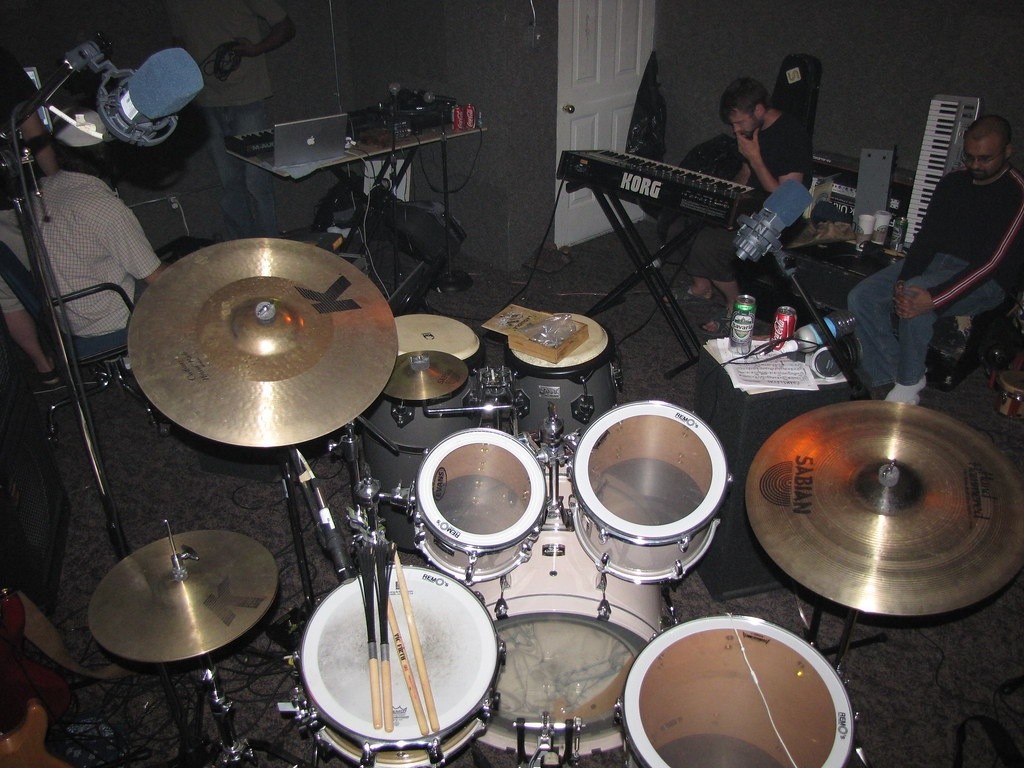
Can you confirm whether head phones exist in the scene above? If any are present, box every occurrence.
[785,332,864,378]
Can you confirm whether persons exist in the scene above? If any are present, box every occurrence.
[165,0,297,239]
[663,77,813,337]
[847,115,1024,406]
[0,46,167,389]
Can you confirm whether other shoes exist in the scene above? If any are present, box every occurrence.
[38,353,60,386]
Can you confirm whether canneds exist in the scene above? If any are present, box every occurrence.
[887,217,909,252]
[769,306,797,350]
[464,104,476,129]
[454,105,463,130]
[729,295,756,355]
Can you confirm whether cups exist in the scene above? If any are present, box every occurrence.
[872,211,891,244]
[856,215,876,251]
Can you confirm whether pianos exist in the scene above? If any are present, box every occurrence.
[553,148,761,369]
[903,93,985,257]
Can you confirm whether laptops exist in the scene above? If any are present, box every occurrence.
[255,113,348,168]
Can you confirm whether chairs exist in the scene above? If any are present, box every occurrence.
[0,240,171,443]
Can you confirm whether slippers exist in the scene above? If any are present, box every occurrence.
[700,315,733,338]
[662,286,716,308]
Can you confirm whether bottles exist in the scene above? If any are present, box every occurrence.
[796,310,859,352]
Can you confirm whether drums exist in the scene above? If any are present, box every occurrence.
[619,611,856,768]
[362,311,483,492]
[415,428,549,583]
[297,563,503,767]
[506,311,621,454]
[569,399,731,585]
[468,460,667,760]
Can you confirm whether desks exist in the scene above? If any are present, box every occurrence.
[226,126,488,291]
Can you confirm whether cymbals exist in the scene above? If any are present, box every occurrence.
[124,235,403,450]
[382,350,469,401]
[86,527,282,665]
[743,400,1023,618]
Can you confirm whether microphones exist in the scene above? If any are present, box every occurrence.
[57,48,203,148]
[389,81,400,123]
[733,179,814,262]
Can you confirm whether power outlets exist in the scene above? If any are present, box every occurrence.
[167,192,179,208]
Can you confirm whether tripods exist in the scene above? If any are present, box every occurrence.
[199,652,315,768]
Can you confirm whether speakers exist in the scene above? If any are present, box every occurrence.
[0,372,72,616]
[691,346,872,602]
[0,305,19,445]
